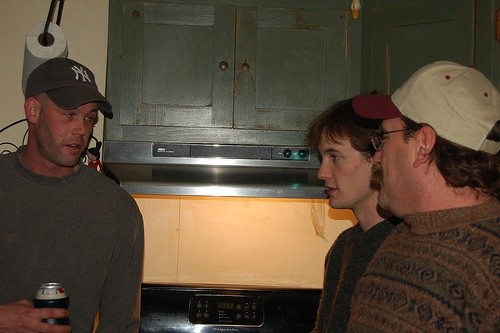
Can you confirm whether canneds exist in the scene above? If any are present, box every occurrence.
[37,282,66,299]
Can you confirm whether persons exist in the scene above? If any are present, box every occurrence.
[0,57,144,333]
[308,92,404,333]
[345,60,500,333]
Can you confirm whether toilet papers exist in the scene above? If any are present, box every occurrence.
[22,21,68,95]
